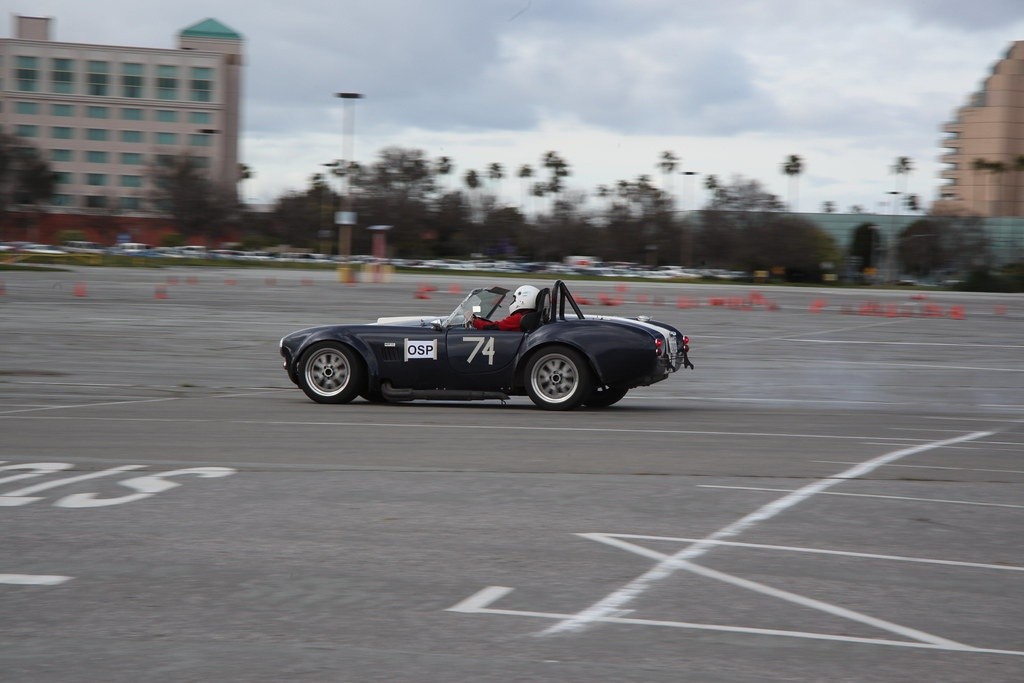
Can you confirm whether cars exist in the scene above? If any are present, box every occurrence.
[0,241,748,283]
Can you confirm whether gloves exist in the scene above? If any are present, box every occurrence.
[464,311,476,326]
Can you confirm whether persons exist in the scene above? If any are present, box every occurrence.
[464,285,540,331]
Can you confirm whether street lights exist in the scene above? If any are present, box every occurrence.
[683,172,697,270]
[335,90,362,258]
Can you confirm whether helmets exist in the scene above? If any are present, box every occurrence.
[508,284,540,316]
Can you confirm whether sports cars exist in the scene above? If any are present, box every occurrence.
[280,278,694,412]
